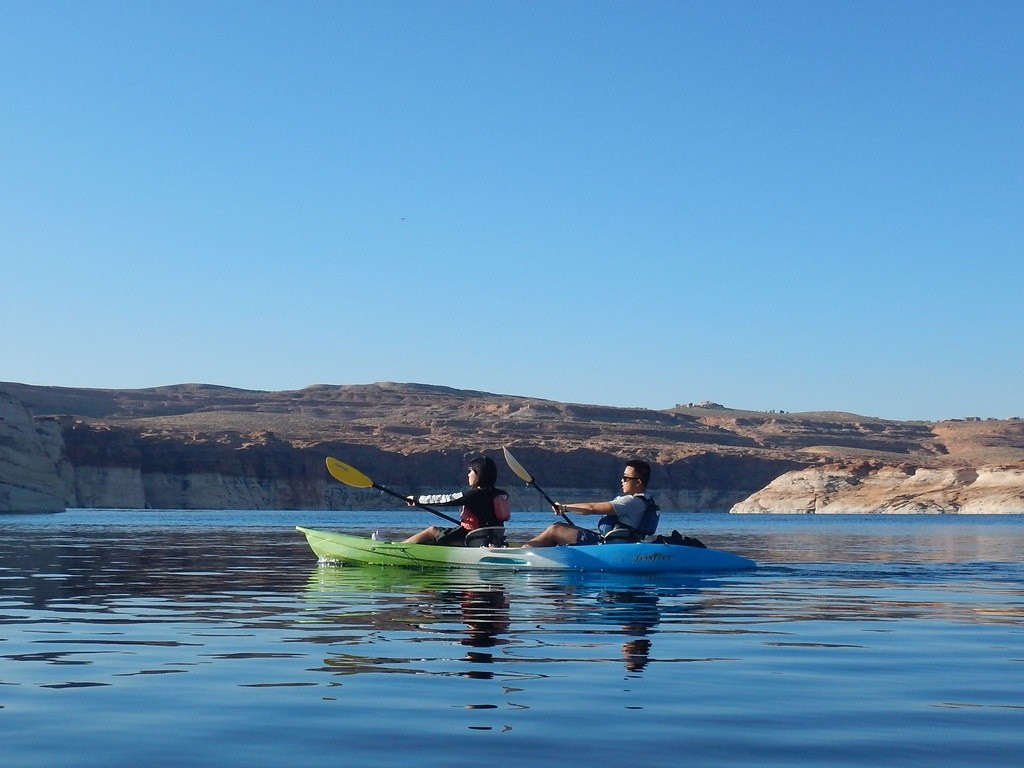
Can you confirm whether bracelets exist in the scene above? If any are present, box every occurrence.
[565,504,567,511]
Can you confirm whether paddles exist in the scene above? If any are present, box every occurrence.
[325,454,464,527]
[501,444,574,525]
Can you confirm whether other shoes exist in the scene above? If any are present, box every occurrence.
[371,530,386,541]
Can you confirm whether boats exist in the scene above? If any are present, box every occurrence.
[295,525,758,572]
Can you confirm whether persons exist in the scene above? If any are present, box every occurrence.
[372,456,505,548]
[521,460,661,548]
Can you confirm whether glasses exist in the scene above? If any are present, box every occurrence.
[621,476,641,482]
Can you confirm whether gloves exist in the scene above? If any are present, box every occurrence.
[552,502,566,516]
